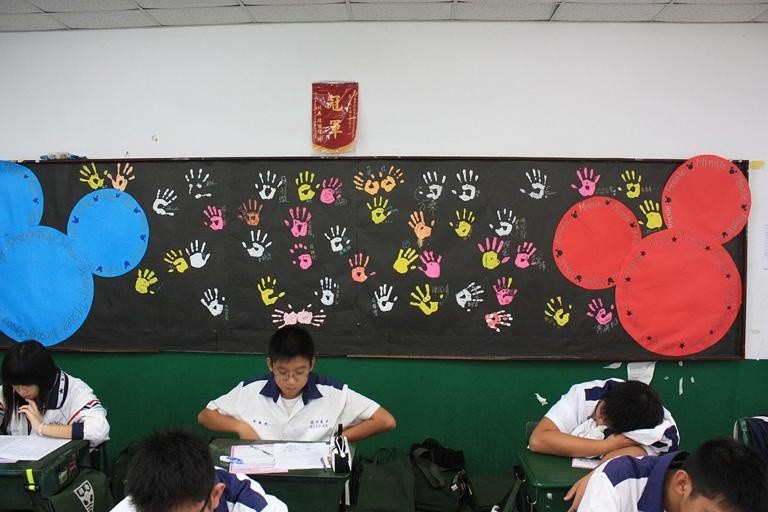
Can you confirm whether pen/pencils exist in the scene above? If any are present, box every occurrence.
[251,446,274,457]
[321,457,328,472]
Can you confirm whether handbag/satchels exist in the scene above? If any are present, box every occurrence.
[23,439,114,512]
[345,447,415,512]
[472,465,520,510]
[412,438,471,511]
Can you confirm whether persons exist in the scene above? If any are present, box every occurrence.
[0,340,110,483]
[110,427,288,512]
[195,324,396,444]
[577,436,768,512]
[528,378,681,511]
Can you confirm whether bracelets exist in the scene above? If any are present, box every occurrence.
[35,422,46,437]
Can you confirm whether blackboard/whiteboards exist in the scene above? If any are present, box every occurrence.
[1,156,750,362]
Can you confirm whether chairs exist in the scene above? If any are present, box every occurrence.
[733,414,768,452]
[525,420,540,444]
